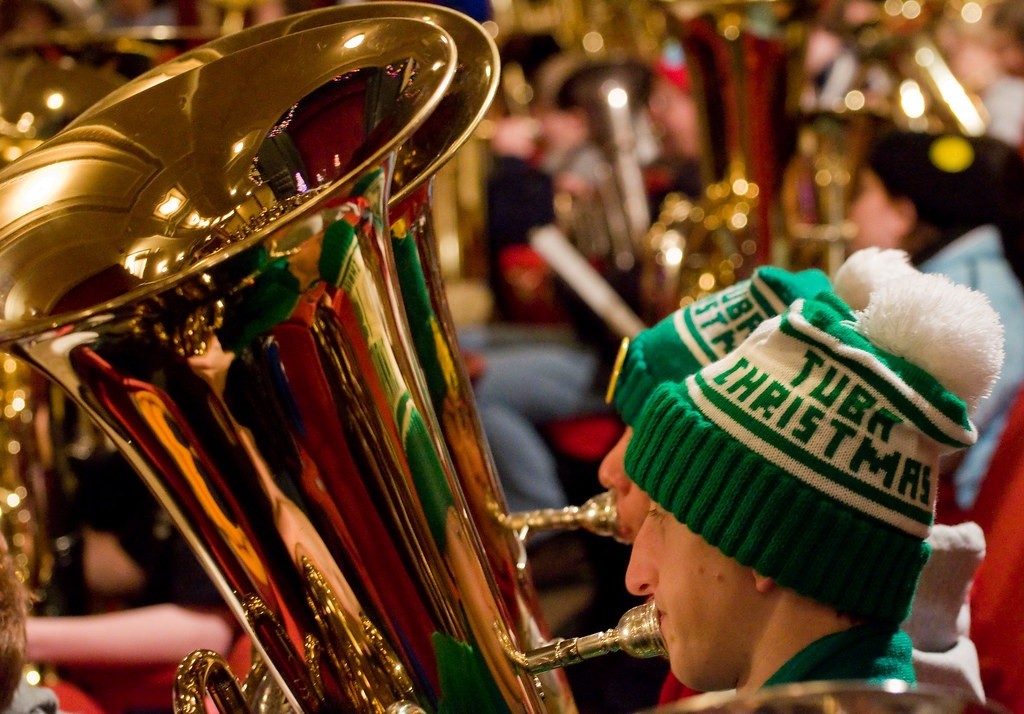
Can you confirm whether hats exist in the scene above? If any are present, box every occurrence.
[606,242,923,432]
[625,268,1006,628]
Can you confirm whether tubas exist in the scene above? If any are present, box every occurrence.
[492,0,987,318]
[1,6,669,714]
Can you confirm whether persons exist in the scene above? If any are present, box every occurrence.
[0,442,254,714]
[448,52,1024,714]
